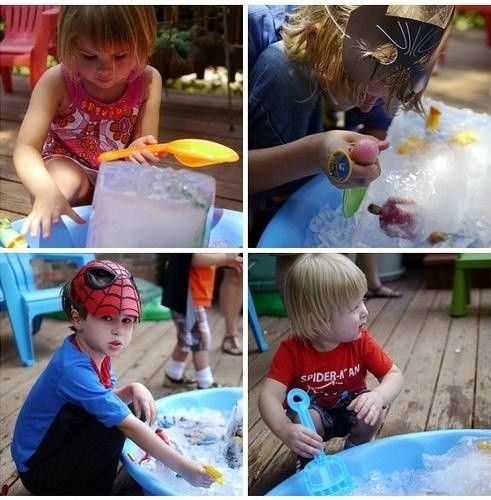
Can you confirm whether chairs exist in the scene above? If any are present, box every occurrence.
[1,253,103,365]
[2,4,66,92]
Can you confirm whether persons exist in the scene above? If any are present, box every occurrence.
[258,253,404,474]
[248,6,453,195]
[269,252,403,301]
[210,254,243,355]
[155,254,243,390]
[13,5,169,240]
[10,259,217,495]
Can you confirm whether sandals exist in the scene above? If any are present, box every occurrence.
[222,335,244,356]
[364,285,403,297]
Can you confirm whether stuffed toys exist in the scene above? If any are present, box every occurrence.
[366,195,420,241]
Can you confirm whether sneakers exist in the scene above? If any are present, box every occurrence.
[163,370,198,389]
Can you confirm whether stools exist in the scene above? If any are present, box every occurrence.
[451,254,491,316]
[249,262,272,352]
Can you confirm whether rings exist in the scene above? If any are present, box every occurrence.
[327,148,351,185]
